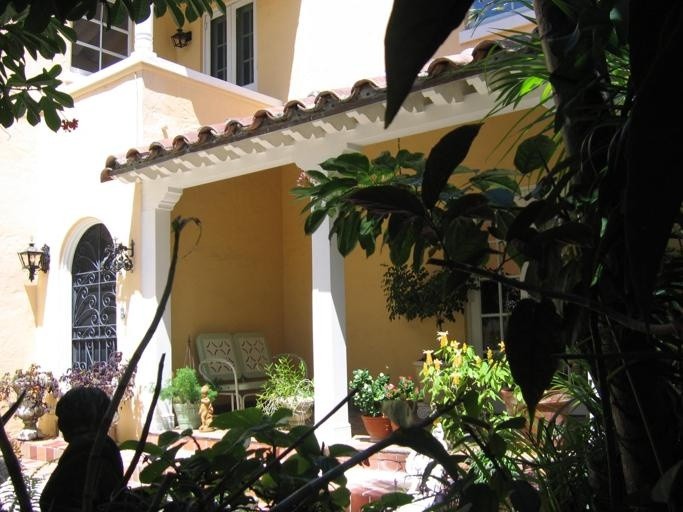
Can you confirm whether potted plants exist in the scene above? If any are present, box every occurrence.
[255,353,314,431]
[149,364,218,429]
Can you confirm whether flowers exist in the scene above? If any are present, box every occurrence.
[385,375,425,402]
[59,352,137,410]
[0,364,63,420]
[348,368,391,417]
[419,330,507,444]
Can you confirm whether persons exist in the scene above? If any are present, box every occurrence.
[38,386,124,511]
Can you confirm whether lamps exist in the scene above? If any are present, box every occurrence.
[171,28,192,48]
[18,236,50,282]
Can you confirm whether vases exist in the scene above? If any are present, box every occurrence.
[17,401,45,441]
[361,414,393,443]
[502,386,571,453]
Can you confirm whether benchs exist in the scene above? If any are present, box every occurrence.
[195,330,308,412]
